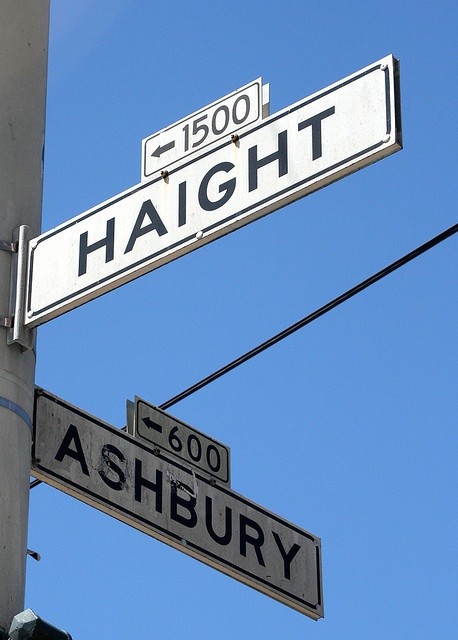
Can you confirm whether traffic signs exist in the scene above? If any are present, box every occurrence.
[30,384,325,621]
[5,53,403,354]
